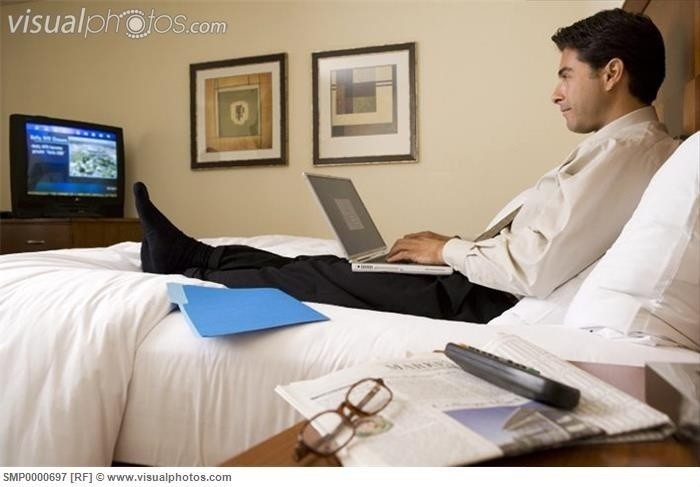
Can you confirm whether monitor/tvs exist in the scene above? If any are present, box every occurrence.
[9,113,127,217]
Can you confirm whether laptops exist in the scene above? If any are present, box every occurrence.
[301,173,454,276]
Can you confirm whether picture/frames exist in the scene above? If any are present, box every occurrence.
[313,41,417,165]
[190,51,288,170]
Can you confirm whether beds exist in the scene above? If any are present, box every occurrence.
[1,236,699,466]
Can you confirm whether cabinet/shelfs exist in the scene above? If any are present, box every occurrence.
[0,218,143,256]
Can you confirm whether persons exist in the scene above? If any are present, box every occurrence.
[133,8,679,324]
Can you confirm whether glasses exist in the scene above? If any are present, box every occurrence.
[290,377,394,463]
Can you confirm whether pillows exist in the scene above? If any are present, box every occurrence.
[485,130,700,353]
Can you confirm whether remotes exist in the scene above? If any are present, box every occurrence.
[443,340,582,410]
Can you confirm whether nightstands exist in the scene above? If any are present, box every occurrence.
[213,361,700,467]
